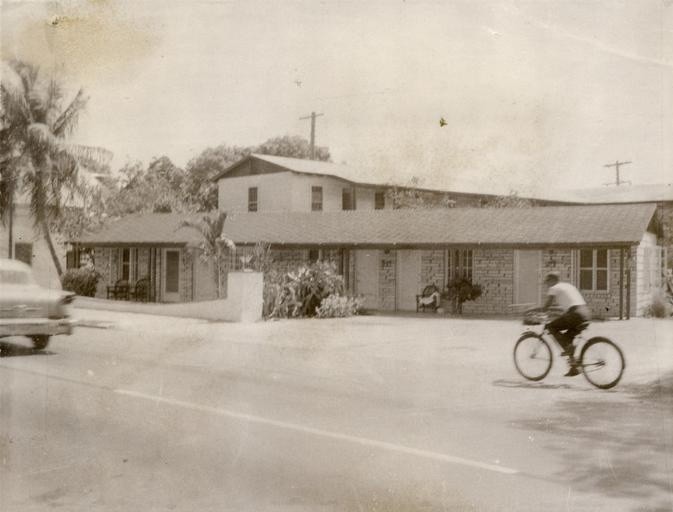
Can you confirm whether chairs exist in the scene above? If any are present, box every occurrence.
[416,282,440,313]
[106,279,128,301]
[129,279,146,301]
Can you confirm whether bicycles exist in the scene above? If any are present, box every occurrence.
[513,308,624,389]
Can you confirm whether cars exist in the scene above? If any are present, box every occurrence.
[0,257,75,350]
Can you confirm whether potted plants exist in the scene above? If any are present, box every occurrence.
[442,277,481,314]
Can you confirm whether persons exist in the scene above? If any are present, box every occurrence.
[525,272,591,376]
